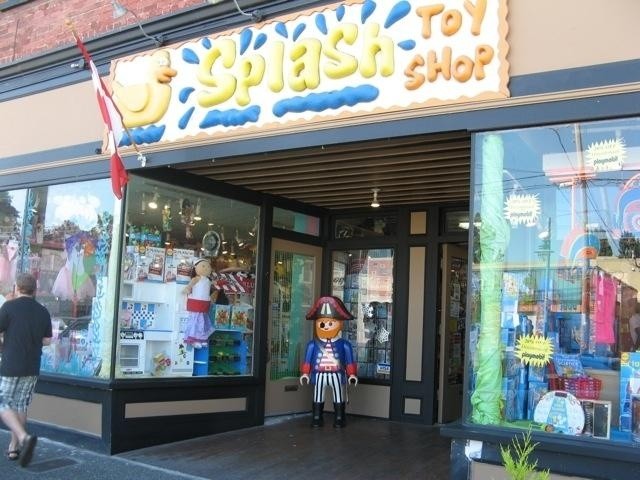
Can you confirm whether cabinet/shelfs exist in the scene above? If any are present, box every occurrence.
[88,246,197,378]
[194,329,246,376]
[474,259,591,358]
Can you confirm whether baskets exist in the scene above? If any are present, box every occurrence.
[557,372,602,401]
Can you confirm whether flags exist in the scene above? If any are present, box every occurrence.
[77,37,128,201]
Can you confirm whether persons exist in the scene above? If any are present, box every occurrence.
[298,295,358,429]
[182,257,215,350]
[0,273,52,468]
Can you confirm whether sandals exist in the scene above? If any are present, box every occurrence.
[7,448,20,461]
[21,432,37,468]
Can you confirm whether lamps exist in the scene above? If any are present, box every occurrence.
[111,0,164,46]
[370,188,381,208]
[457,213,481,229]
[204,0,263,23]
[123,187,285,268]
